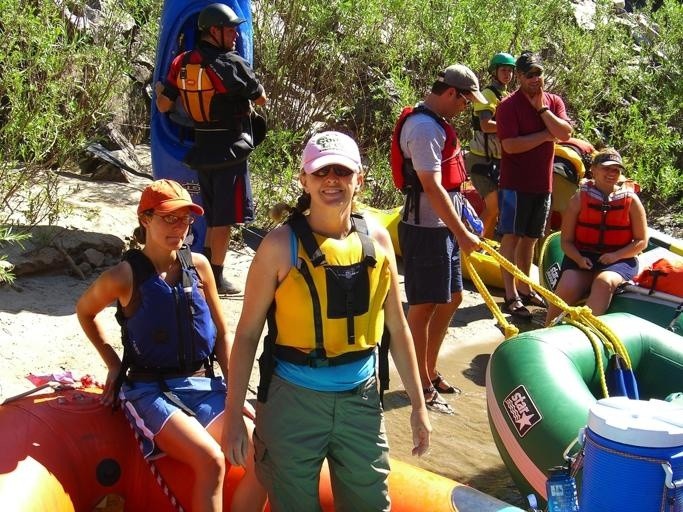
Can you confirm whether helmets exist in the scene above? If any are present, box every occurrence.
[490,51,515,77]
[196,3,247,31]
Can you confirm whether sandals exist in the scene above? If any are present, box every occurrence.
[431,371,460,397]
[413,386,454,417]
[519,290,548,307]
[503,297,532,319]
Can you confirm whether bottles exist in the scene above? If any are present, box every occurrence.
[545,463,580,511]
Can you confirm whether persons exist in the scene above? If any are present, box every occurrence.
[392,66,487,415]
[546,147,649,327]
[546,139,597,231]
[471,52,516,239]
[496,53,572,320]
[76,178,267,511]
[154,5,267,295]
[221,132,431,512]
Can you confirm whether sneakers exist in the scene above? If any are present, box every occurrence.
[210,281,241,295]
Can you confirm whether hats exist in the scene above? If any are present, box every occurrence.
[303,131,360,175]
[592,148,625,171]
[515,53,543,72]
[137,178,203,221]
[437,66,488,106]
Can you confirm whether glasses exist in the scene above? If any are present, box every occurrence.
[520,70,543,80]
[310,164,353,178]
[150,213,194,227]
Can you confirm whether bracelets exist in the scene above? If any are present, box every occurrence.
[537,107,549,115]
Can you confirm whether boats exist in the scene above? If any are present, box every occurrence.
[145,0,254,274]
[0,367,530,511]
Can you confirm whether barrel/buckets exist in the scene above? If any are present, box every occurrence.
[577,394,683,512]
